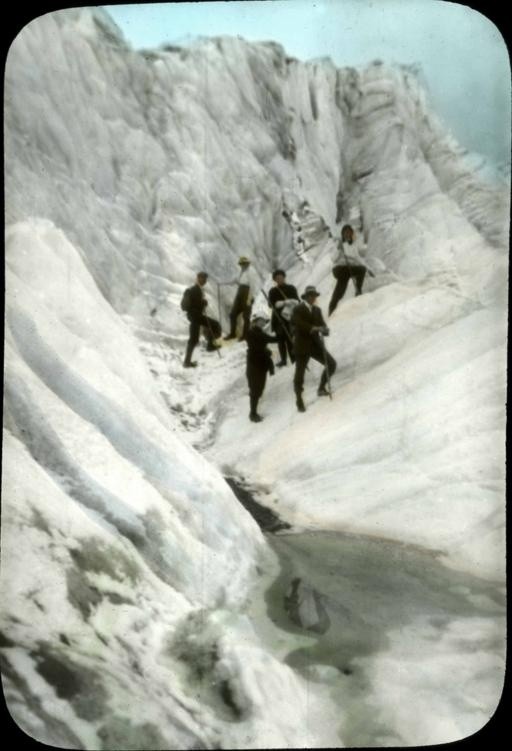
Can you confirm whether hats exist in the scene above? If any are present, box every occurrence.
[272,270,286,280]
[252,312,270,323]
[337,222,358,242]
[238,257,251,264]
[301,285,320,300]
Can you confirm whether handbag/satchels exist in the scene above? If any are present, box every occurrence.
[333,265,344,279]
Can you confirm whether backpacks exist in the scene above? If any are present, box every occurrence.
[181,289,191,310]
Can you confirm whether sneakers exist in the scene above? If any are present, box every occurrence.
[276,360,286,366]
[318,390,331,396]
[207,333,247,352]
[249,412,262,422]
[296,399,306,413]
[183,362,197,367]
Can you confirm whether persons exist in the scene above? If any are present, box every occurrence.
[180,224,375,421]
